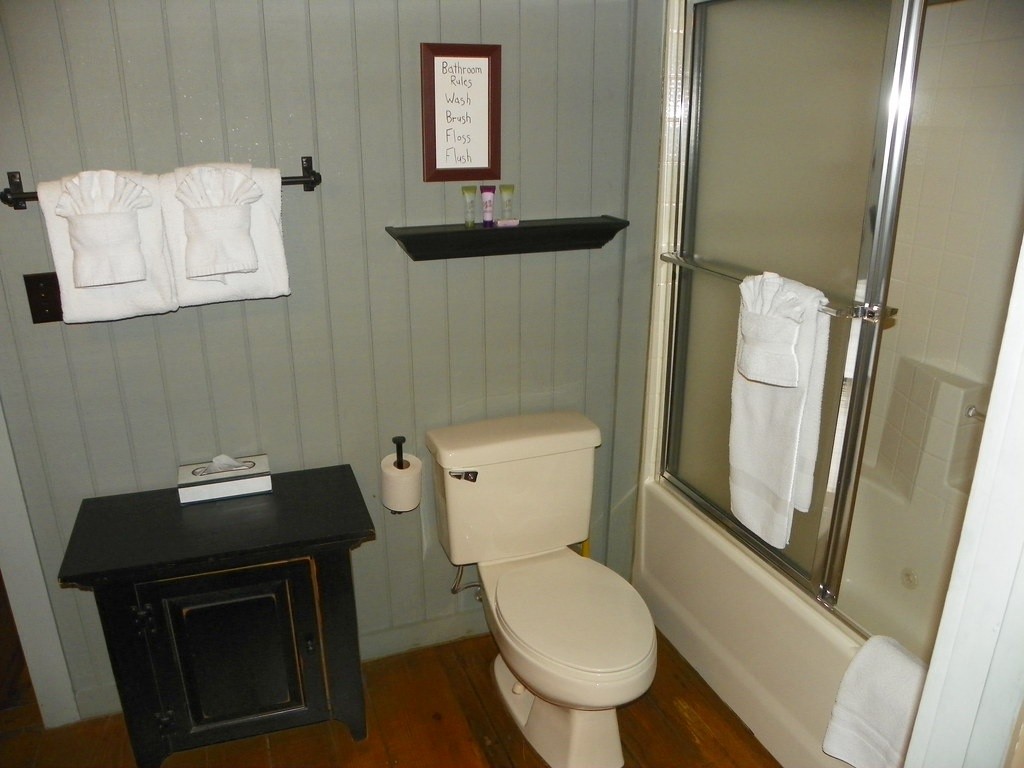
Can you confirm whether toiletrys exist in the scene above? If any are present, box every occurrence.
[462,183,514,229]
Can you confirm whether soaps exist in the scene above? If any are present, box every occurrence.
[494,218,519,228]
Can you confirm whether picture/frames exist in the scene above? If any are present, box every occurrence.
[420,42,501,181]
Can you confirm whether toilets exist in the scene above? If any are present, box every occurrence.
[425,411,657,768]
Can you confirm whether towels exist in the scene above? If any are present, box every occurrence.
[821,634,929,768]
[39,163,291,324]
[728,271,832,555]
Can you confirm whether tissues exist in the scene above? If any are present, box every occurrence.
[175,453,275,505]
[381,452,423,512]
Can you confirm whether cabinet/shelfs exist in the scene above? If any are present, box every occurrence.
[94,543,367,767]
